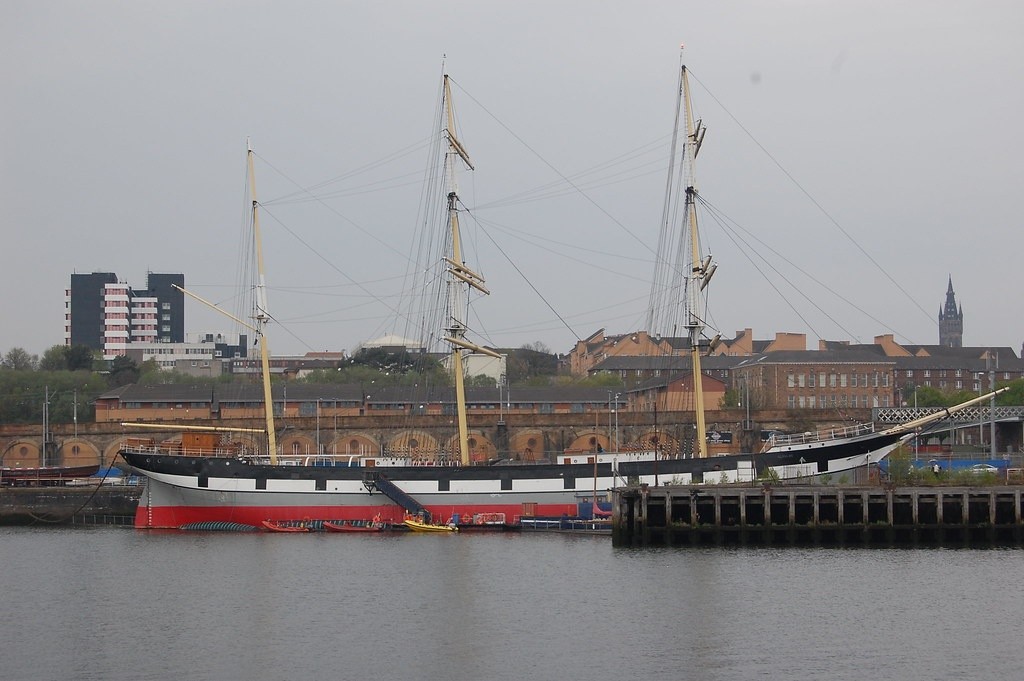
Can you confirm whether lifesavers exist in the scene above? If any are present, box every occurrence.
[373,516,380,522]
[461,514,471,523]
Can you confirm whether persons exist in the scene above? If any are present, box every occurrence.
[372,512,381,528]
[406,513,453,526]
[805,430,811,443]
[932,463,940,476]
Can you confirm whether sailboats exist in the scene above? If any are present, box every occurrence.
[119,43,1011,533]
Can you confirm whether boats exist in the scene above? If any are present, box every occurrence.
[0,464,100,481]
